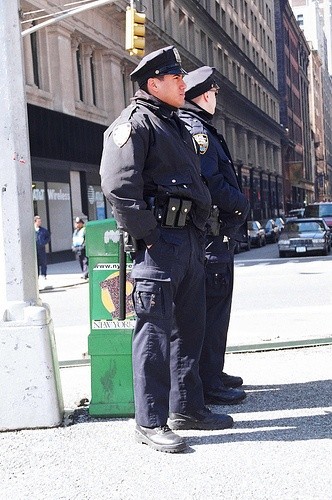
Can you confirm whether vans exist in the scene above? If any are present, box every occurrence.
[302,200,332,234]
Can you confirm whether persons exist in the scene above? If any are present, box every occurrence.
[178,66,250,407]
[34,215,51,280]
[100,44,234,453]
[71,217,89,280]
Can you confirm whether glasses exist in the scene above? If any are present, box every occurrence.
[209,89,219,95]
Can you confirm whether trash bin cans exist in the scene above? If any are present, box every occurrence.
[85,219,138,418]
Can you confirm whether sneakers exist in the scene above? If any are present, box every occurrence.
[135,425,186,453]
[167,407,234,430]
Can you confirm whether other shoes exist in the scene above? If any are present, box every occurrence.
[219,371,244,388]
[204,386,246,404]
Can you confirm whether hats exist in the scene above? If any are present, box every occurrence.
[75,217,84,224]
[130,44,189,86]
[182,66,220,99]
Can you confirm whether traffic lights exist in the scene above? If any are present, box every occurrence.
[125,9,147,56]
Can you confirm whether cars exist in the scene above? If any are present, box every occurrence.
[234,209,305,254]
[277,217,332,258]
